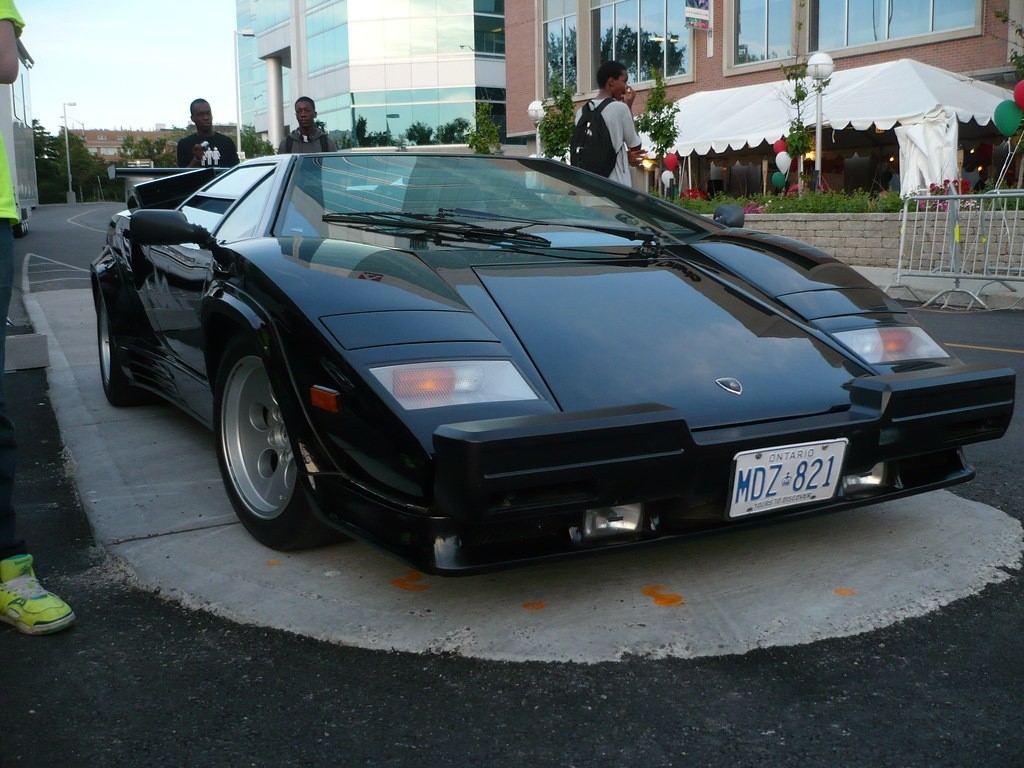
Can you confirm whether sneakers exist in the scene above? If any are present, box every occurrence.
[0,553,76,637]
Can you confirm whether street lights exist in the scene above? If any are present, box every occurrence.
[807,51,835,191]
[63,103,77,204]
[526,100,548,159]
[234,28,258,155]
[60,116,85,148]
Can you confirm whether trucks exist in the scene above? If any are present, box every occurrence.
[0,38,40,238]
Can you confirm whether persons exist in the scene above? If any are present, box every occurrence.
[277,96,336,155]
[177,99,240,168]
[574,61,648,188]
[0,0,76,636]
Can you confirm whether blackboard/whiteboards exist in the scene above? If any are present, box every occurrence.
[708,180,723,201]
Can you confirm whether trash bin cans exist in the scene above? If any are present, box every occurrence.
[667,187,678,202]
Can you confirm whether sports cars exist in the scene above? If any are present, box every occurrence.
[88,148,1019,579]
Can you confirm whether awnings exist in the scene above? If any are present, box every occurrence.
[634,58,1015,160]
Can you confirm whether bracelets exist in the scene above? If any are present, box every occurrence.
[193,155,200,162]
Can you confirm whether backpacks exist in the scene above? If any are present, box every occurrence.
[569,98,624,178]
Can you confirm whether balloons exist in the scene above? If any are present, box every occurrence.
[772,172,785,189]
[1014,80,1024,111]
[773,140,787,154]
[662,170,674,185]
[665,153,677,170]
[993,99,1022,138]
[776,151,792,174]
